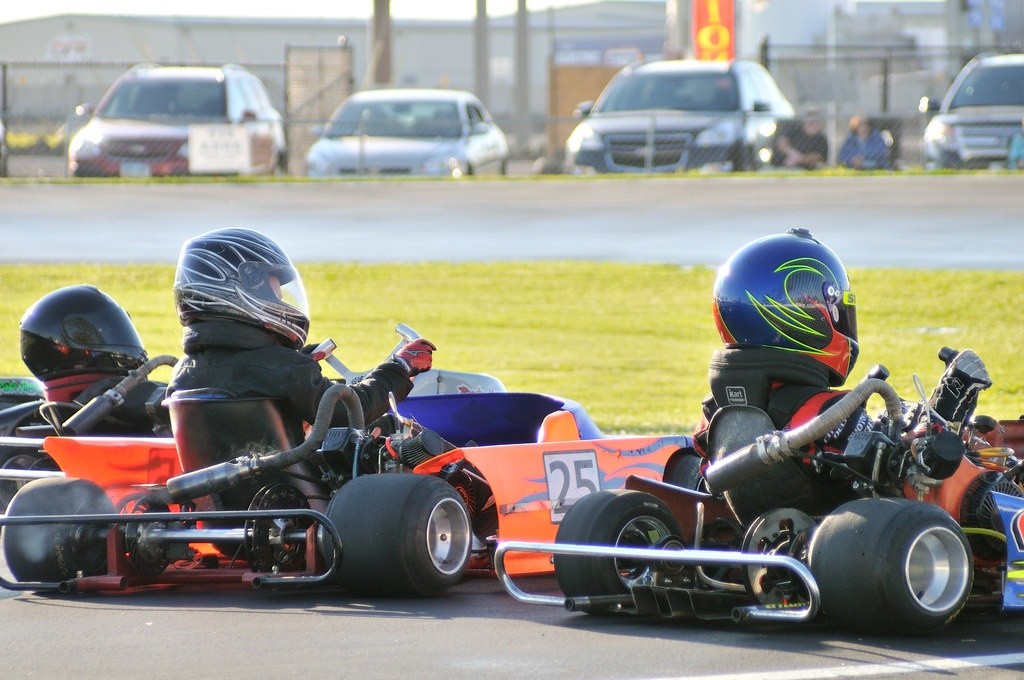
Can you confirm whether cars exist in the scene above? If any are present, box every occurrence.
[304,88,511,179]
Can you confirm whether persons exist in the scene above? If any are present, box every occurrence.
[770,106,892,170]
[695,230,992,463]
[20,285,169,436]
[166,227,437,447]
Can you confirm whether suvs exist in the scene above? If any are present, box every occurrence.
[917,52,1023,172]
[66,62,288,177]
[563,56,793,176]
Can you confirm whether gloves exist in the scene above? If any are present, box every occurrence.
[390,339,437,377]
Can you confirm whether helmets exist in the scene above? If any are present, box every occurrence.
[174,228,308,350]
[712,228,860,388]
[18,284,147,399]
